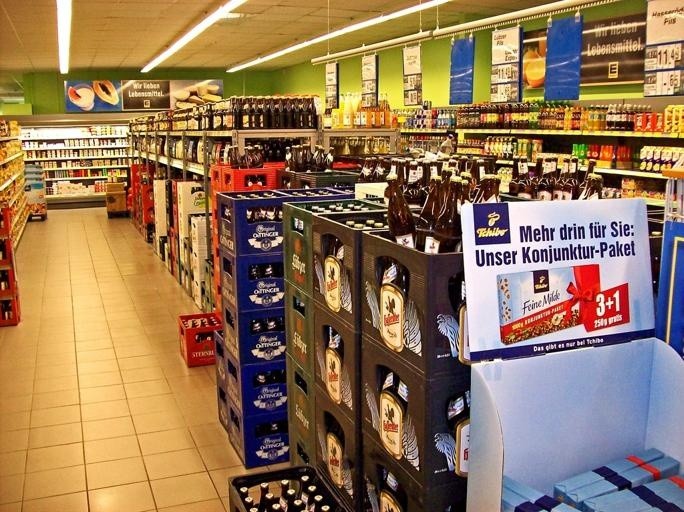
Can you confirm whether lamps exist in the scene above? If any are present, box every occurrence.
[55,1,73,76]
[225,0,452,73]
[140,0,247,74]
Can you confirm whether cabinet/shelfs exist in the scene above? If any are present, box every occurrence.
[126,130,318,181]
[0,119,32,258]
[325,129,448,166]
[448,100,684,231]
[20,120,130,208]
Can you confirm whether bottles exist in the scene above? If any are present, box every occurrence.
[451,109,456,128]
[285,145,334,185]
[593,105,601,130]
[445,109,452,128]
[563,106,571,130]
[224,99,230,129]
[635,104,652,114]
[491,101,498,128]
[510,101,519,128]
[264,97,270,129]
[549,104,556,129]
[605,103,613,130]
[301,98,310,129]
[462,104,468,127]
[294,98,300,128]
[269,97,277,128]
[468,102,474,128]
[486,102,492,128]
[202,106,206,130]
[542,102,549,129]
[250,98,256,128]
[360,155,492,182]
[285,98,292,128]
[0,210,18,326]
[193,106,199,130]
[510,156,520,196]
[206,103,213,130]
[615,103,621,130]
[498,102,505,129]
[236,96,246,130]
[278,98,284,128]
[556,105,564,131]
[429,178,461,254]
[579,160,601,200]
[529,99,540,129]
[228,145,264,187]
[480,101,486,127]
[556,158,568,202]
[455,104,462,127]
[550,156,559,203]
[229,97,237,130]
[353,93,362,128]
[418,177,444,251]
[519,101,530,129]
[518,156,530,200]
[530,157,542,199]
[387,175,416,249]
[572,108,580,129]
[474,102,479,128]
[310,98,317,129]
[580,105,588,129]
[344,92,353,129]
[563,159,577,201]
[372,93,380,128]
[621,103,627,129]
[222,192,469,511]
[256,97,264,128]
[504,101,511,128]
[588,105,594,130]
[627,103,634,130]
[437,109,442,128]
[441,108,447,129]
[182,317,218,363]
[214,98,223,130]
[437,134,453,156]
[537,158,553,201]
[333,137,387,155]
[361,93,372,127]
[198,105,203,130]
[611,104,617,130]
[381,92,390,127]
[242,98,250,129]
[172,108,195,130]
